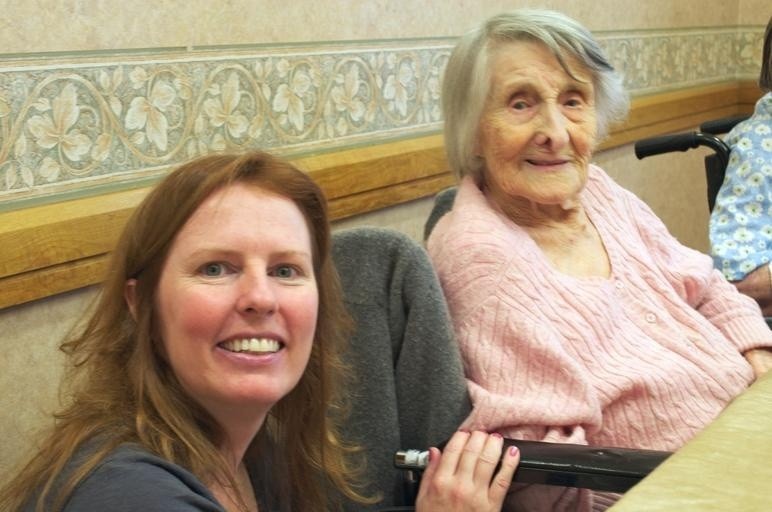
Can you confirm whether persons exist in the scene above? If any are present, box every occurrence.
[427,9,771,511]
[708,16,772,329]
[0,150,522,512]
